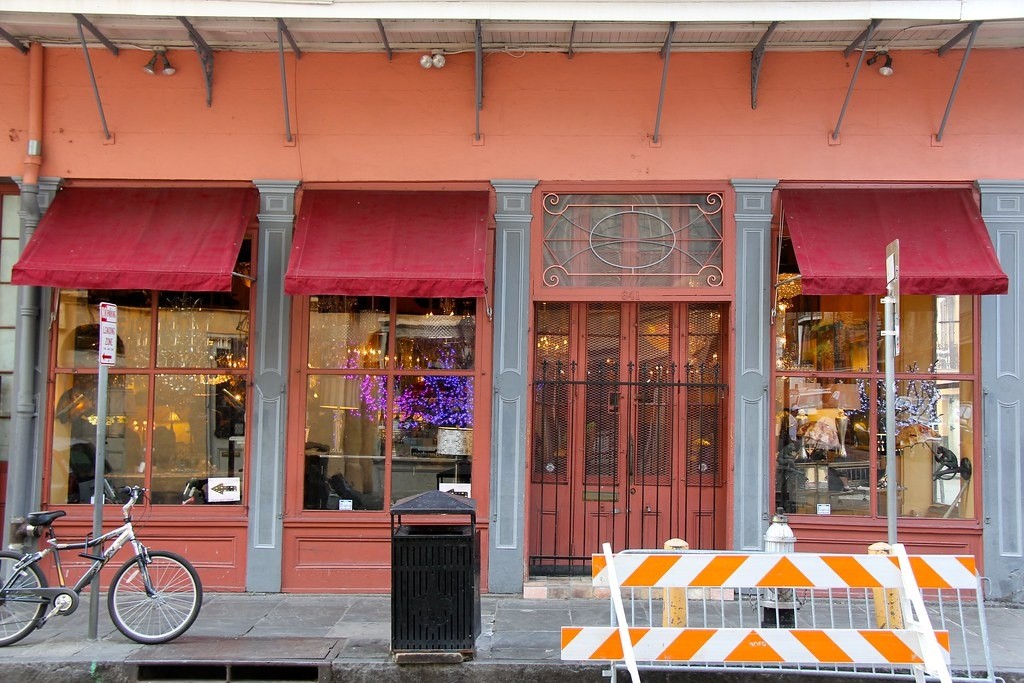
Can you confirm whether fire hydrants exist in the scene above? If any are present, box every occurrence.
[752,507,804,628]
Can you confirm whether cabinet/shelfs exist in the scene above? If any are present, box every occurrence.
[879,440,932,518]
[794,461,829,514]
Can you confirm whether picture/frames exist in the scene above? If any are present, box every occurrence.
[815,322,839,383]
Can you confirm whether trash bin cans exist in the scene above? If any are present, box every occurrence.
[389,491,481,661]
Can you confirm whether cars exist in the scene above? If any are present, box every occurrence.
[792,391,846,436]
[960,399,973,441]
[834,408,870,449]
[775,443,881,514]
[52,437,114,504]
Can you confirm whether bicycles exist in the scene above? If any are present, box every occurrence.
[0,484,203,648]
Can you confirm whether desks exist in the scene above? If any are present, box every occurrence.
[370,454,472,505]
[436,464,471,489]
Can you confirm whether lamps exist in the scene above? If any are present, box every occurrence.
[775,298,795,311]
[131,415,156,444]
[895,421,971,481]
[319,376,361,454]
[776,264,802,301]
[436,424,473,482]
[537,333,569,360]
[821,382,862,458]
[132,288,247,414]
[161,406,180,430]
